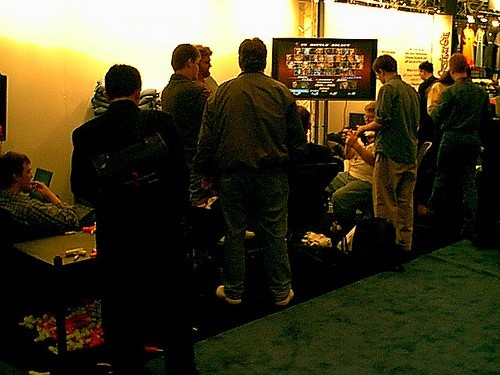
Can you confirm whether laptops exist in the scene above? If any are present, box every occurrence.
[32,167,52,187]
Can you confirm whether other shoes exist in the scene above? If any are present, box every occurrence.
[216,230,256,246]
[276,289,295,306]
[216,285,242,305]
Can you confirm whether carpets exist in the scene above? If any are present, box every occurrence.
[145,239,500,375]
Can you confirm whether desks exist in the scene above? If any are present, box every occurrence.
[13,226,106,375]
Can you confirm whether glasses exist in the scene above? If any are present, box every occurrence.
[363,114,375,120]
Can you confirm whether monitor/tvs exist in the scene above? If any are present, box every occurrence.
[272,37,377,101]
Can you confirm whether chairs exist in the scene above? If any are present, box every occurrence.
[416,141,432,184]
[244,162,344,267]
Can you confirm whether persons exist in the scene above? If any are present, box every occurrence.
[161,44,218,116]
[356,56,420,255]
[0,152,79,243]
[288,101,375,220]
[71,65,197,375]
[195,39,308,305]
[416,54,490,237]
[418,61,451,114]
